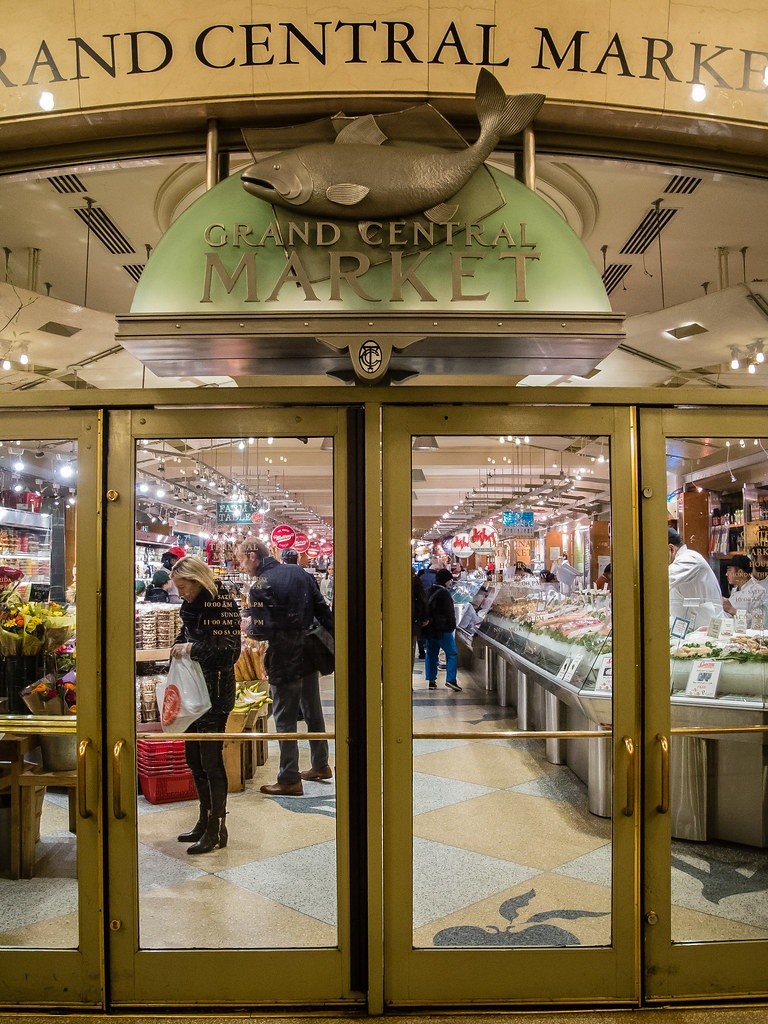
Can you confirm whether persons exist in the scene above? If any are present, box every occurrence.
[65,565,76,603]
[170,557,242,855]
[596,564,610,590]
[540,570,560,589]
[0,583,27,607]
[487,562,495,575]
[320,563,334,612]
[514,561,532,575]
[412,558,465,691]
[135,547,187,604]
[236,536,335,795]
[668,528,768,631]
[281,548,298,564]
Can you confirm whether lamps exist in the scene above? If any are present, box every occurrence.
[195,461,229,497]
[413,436,439,450]
[3,346,28,370]
[730,338,764,374]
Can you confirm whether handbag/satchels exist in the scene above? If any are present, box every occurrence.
[304,623,335,676]
[155,642,212,733]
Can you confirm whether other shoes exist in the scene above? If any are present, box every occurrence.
[445,682,462,691]
[429,682,437,689]
[438,664,446,671]
[419,654,426,661]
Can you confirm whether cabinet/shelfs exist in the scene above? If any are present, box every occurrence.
[707,483,768,581]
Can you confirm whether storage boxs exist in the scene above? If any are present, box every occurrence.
[136,603,184,650]
[134,676,165,723]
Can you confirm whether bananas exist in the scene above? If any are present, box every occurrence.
[231,681,273,713]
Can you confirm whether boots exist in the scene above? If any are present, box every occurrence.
[178,804,212,842]
[187,813,229,854]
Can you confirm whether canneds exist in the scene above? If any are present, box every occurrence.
[208,566,256,580]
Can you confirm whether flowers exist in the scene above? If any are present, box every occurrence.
[0,583,77,716]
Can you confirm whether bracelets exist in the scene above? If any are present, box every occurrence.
[181,643,190,658]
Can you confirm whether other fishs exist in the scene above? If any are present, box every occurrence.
[240,64,547,226]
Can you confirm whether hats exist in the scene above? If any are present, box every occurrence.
[668,527,677,539]
[135,580,146,593]
[436,569,453,582]
[722,554,753,574]
[169,547,185,559]
[153,571,170,583]
[545,573,554,583]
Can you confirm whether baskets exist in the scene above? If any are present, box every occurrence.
[137,738,200,805]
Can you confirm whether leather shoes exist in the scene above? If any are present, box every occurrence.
[301,766,332,780]
[260,782,304,795]
